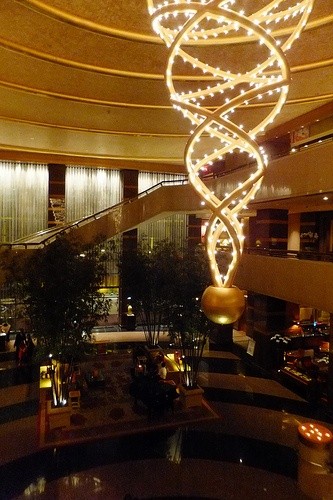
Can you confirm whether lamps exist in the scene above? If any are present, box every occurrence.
[144,0,316,326]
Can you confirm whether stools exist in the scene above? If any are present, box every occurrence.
[64,343,141,411]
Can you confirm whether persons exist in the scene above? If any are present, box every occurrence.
[301,356,315,380]
[154,363,167,385]
[14,328,34,368]
[302,324,328,334]
[290,356,303,373]
[154,351,164,365]
[314,349,322,359]
